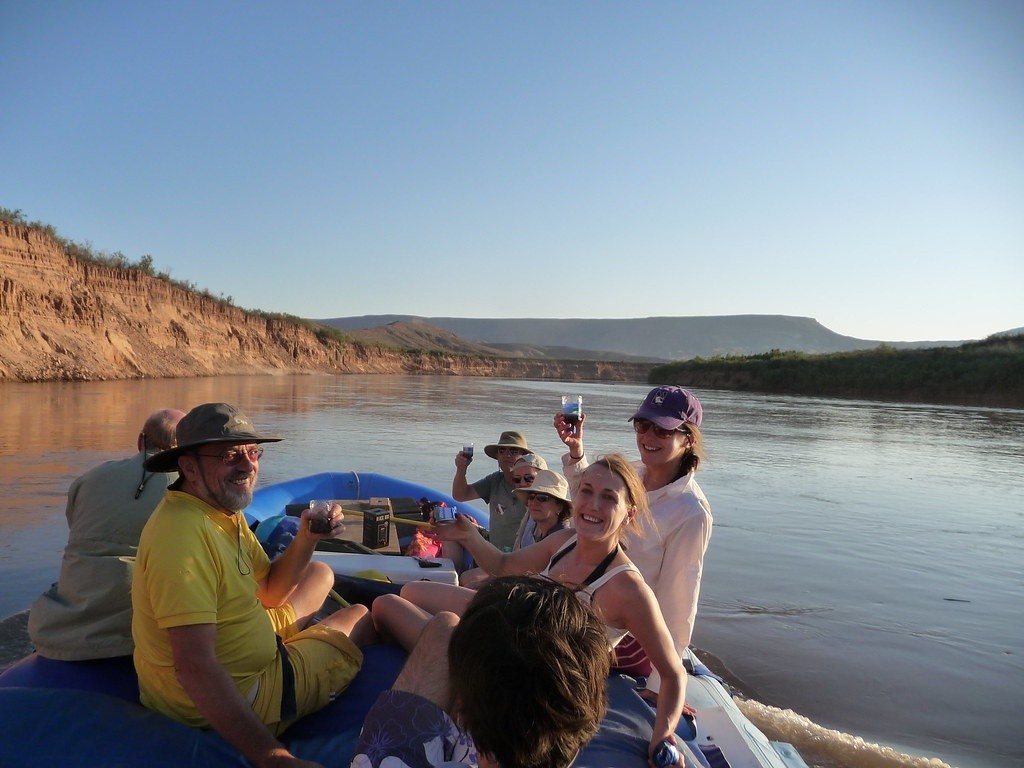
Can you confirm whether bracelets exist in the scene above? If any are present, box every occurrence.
[570,452,584,459]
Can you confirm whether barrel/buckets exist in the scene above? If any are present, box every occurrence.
[363,509,391,549]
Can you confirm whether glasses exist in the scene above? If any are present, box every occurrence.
[199,447,263,466]
[634,418,689,439]
[529,493,554,502]
[512,474,535,483]
[499,447,518,454]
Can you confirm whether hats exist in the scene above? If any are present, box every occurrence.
[628,385,703,430]
[510,454,548,471]
[143,402,285,473]
[484,431,534,460]
[511,470,571,502]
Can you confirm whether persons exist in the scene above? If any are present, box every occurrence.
[27,406,190,663]
[370,455,689,768]
[460,469,575,587]
[511,454,554,554]
[345,574,609,768]
[130,402,372,767]
[440,430,537,585]
[554,384,714,717]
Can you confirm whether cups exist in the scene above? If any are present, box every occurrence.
[310,499,332,533]
[463,442,474,462]
[562,395,582,434]
